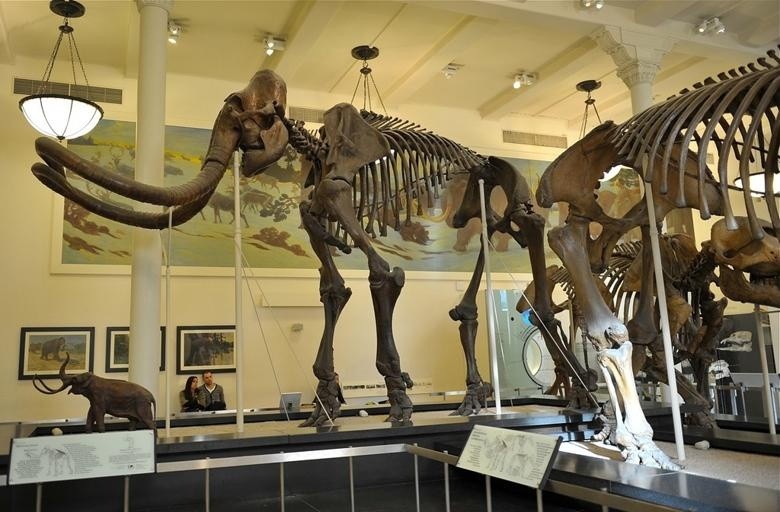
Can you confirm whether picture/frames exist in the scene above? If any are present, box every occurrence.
[15,325,97,381]
[175,323,240,375]
[106,323,168,375]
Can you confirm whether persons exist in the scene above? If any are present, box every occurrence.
[178,376,200,412]
[197,370,226,411]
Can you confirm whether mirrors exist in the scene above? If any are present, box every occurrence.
[524,328,569,395]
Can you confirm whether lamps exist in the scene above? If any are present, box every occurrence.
[570,79,626,186]
[341,43,401,170]
[18,1,106,147]
[730,122,780,203]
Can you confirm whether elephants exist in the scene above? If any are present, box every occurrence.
[32,351,156,434]
